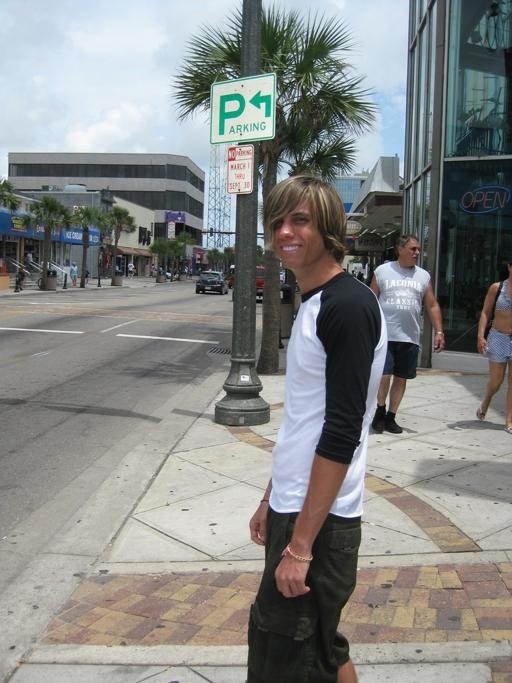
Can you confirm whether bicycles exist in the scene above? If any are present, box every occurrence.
[37,272,43,287]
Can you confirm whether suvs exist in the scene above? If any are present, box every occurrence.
[193,269,229,295]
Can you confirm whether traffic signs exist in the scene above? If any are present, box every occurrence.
[208,72,277,143]
[227,142,255,195]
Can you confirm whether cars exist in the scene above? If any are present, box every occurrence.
[255,264,264,298]
[280,271,285,282]
[228,264,235,288]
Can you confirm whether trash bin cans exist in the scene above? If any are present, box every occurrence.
[111,269,123,285]
[156,270,166,283]
[44,269,57,290]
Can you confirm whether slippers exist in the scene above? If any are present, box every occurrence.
[504,426,512,435]
[476,406,486,421]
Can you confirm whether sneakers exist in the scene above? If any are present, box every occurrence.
[384,415,403,434]
[372,408,385,431]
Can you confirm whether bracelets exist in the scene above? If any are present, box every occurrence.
[287,542,313,562]
[435,332,445,337]
[261,500,268,502]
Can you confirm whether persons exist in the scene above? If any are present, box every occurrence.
[27,251,33,271]
[370,235,446,434]
[246,175,388,683]
[476,260,512,434]
[70,261,79,286]
[127,263,194,281]
[17,267,25,290]
